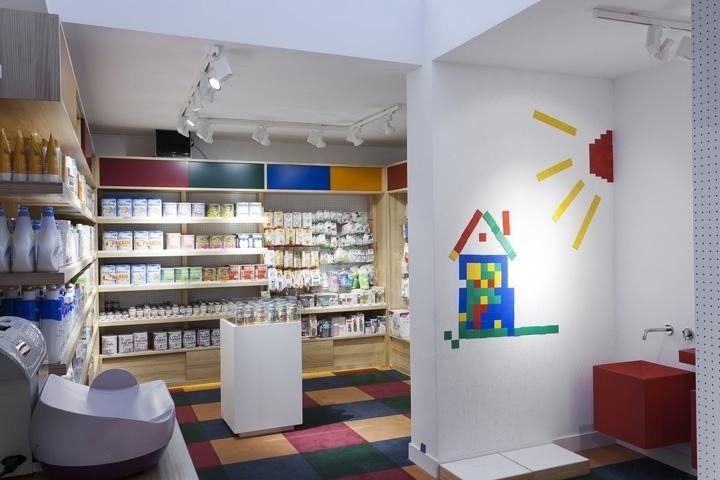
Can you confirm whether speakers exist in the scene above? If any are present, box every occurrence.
[155,129,192,158]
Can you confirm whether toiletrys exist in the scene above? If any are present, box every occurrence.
[1,127,61,183]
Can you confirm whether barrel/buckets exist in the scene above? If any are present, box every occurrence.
[36,206,64,273]
[0,205,12,274]
[11,204,36,271]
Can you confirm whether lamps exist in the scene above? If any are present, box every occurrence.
[595,12,694,71]
[195,117,347,146]
[345,103,399,146]
[177,41,232,138]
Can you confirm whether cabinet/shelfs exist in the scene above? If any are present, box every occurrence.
[1,99,100,390]
[99,182,392,389]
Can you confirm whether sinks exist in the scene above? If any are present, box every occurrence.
[590,359,695,448]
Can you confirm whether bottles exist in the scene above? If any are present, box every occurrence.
[223,302,298,325]
[99,300,223,320]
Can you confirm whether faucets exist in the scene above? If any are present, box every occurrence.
[641,324,673,340]
